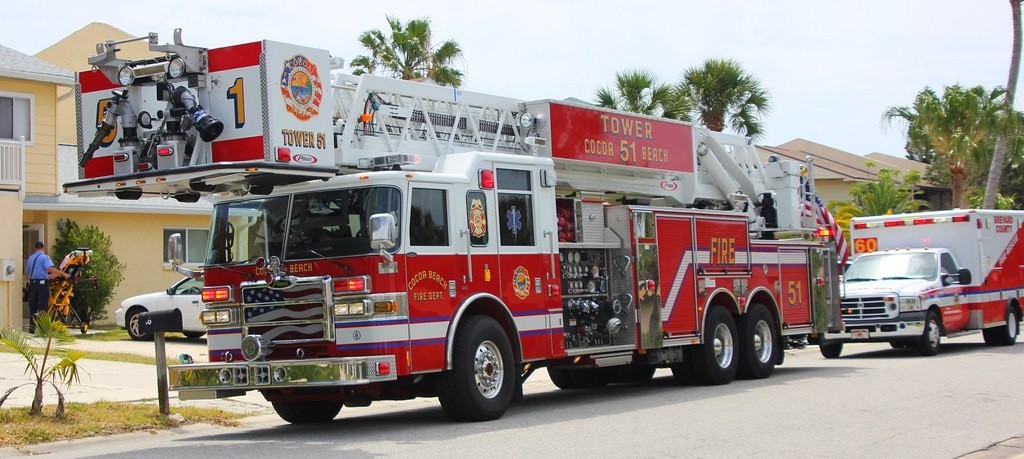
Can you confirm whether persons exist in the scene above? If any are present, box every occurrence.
[26,241,71,334]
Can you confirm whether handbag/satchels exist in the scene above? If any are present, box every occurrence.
[23,281,30,293]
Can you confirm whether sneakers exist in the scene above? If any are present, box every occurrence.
[40,327,48,334]
[29,324,35,333]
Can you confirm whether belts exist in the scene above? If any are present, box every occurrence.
[31,279,48,284]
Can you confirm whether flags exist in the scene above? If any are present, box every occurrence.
[800,175,850,265]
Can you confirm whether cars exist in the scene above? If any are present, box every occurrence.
[115,270,207,342]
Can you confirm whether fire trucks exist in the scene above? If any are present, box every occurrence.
[817,208,1021,359]
[60,28,850,426]
